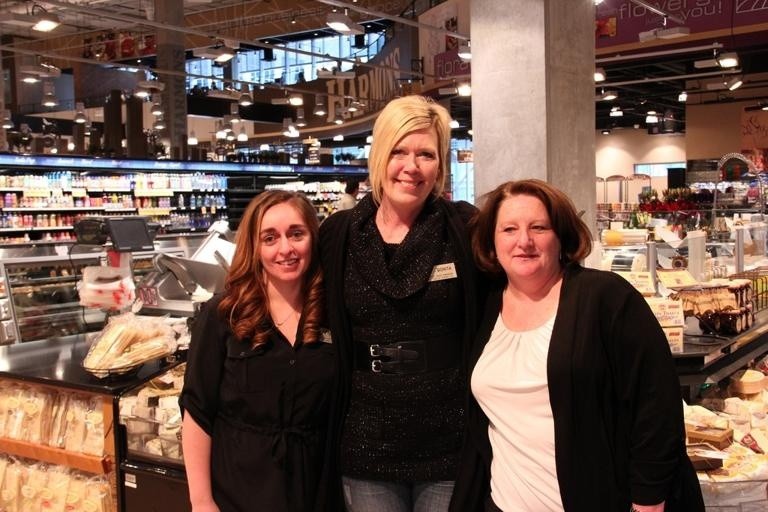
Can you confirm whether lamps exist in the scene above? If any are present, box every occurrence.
[327,12,365,36]
[716,52,744,91]
[190,45,236,63]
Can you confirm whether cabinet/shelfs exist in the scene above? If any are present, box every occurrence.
[0,328,192,511]
[0,152,369,351]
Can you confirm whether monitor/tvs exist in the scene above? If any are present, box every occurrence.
[106,217,155,251]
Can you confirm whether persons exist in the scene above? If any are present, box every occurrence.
[80,29,157,61]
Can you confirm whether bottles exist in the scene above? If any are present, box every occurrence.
[0,171,228,246]
[698,284,754,335]
